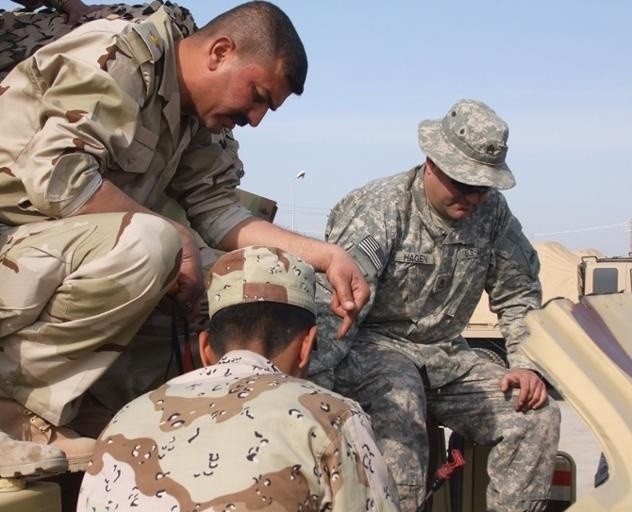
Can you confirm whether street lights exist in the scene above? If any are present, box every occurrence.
[289,169,306,232]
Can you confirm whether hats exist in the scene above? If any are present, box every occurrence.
[416,98,516,193]
[206,243,319,351]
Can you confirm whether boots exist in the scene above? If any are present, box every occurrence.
[0,393,98,480]
[0,431,70,484]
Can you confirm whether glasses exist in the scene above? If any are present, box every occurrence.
[448,181,494,196]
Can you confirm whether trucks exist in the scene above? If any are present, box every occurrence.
[409,231,632,397]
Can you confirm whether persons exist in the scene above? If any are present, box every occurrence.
[77,246,402,511]
[311,99,562,511]
[0,13,372,482]
